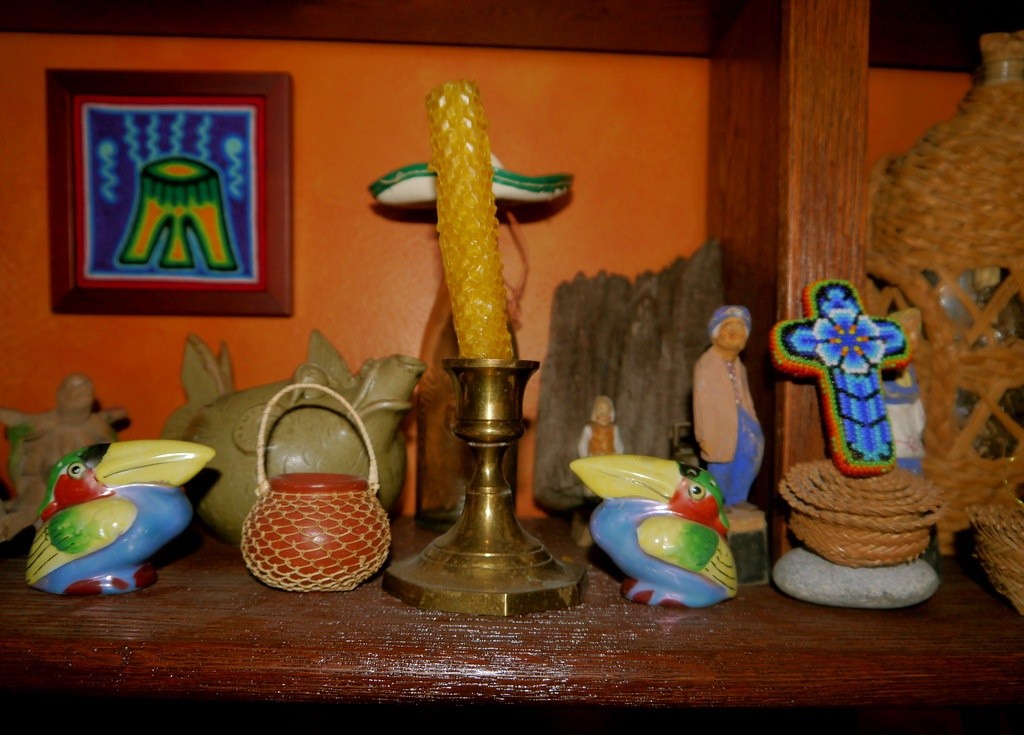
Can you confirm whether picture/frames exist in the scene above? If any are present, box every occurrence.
[41,67,297,319]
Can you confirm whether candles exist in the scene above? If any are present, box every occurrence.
[426,76,515,358]
